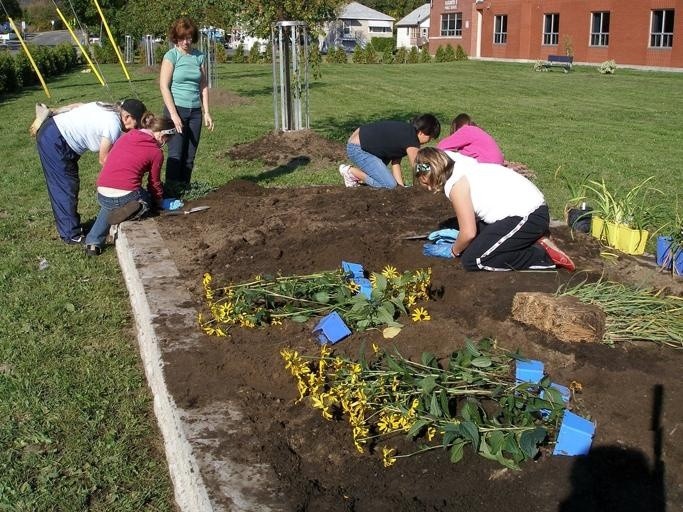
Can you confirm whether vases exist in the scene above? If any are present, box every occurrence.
[311,311,352,347]
[342,261,375,300]
[513,359,596,458]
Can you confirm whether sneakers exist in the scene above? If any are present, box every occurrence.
[68,227,89,244]
[338,164,361,188]
[106,201,142,225]
[88,244,100,255]
[537,235,575,272]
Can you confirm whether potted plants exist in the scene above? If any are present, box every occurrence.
[603,176,654,247]
[618,188,665,256]
[570,177,622,239]
[554,166,598,233]
[649,194,683,277]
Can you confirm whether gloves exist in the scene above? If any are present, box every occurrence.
[423,228,459,260]
[161,198,184,210]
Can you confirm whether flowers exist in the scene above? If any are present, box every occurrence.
[197,271,357,337]
[342,264,433,331]
[278,347,558,469]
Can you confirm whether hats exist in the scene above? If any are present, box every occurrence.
[124,99,146,129]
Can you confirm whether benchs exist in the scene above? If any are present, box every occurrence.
[541,55,573,73]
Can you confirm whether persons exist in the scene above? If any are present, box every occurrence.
[158,17,216,210]
[436,113,504,164]
[413,147,576,273]
[35,99,146,244]
[339,115,440,190]
[81,112,175,255]
